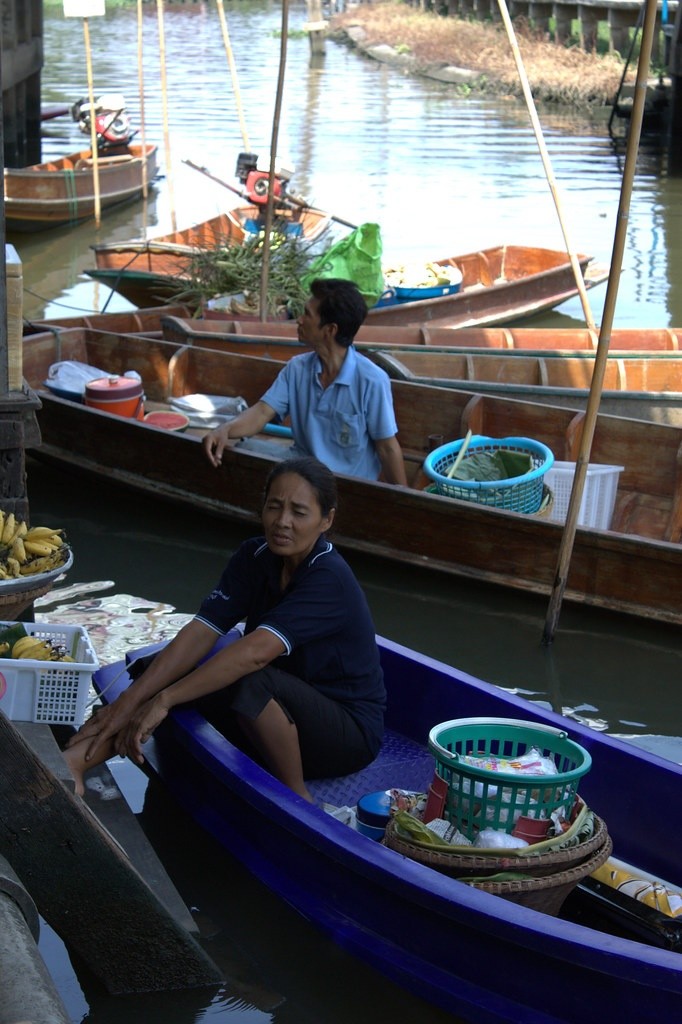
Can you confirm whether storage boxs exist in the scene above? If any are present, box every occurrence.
[422,432,555,515]
[385,265,465,304]
[0,540,75,607]
[384,715,616,917]
[421,482,554,521]
[529,457,626,532]
[0,620,101,726]
[81,374,147,423]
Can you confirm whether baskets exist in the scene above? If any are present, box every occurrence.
[1,620,99,724]
[423,483,554,519]
[375,291,403,308]
[0,581,52,622]
[422,433,554,514]
[379,718,613,919]
[383,265,464,299]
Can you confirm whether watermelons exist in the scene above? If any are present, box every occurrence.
[143,411,189,433]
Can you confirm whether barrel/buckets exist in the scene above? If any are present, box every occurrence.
[387,266,463,303]
[85,374,144,422]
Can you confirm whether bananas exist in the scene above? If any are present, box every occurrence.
[0,508,78,662]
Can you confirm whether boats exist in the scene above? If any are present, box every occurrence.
[18,327,682,636]
[19,238,624,344]
[1,141,159,226]
[81,207,335,310]
[158,313,682,366]
[89,628,682,1024]
[363,345,682,434]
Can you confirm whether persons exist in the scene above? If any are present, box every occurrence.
[60,457,389,804]
[202,276,409,489]
